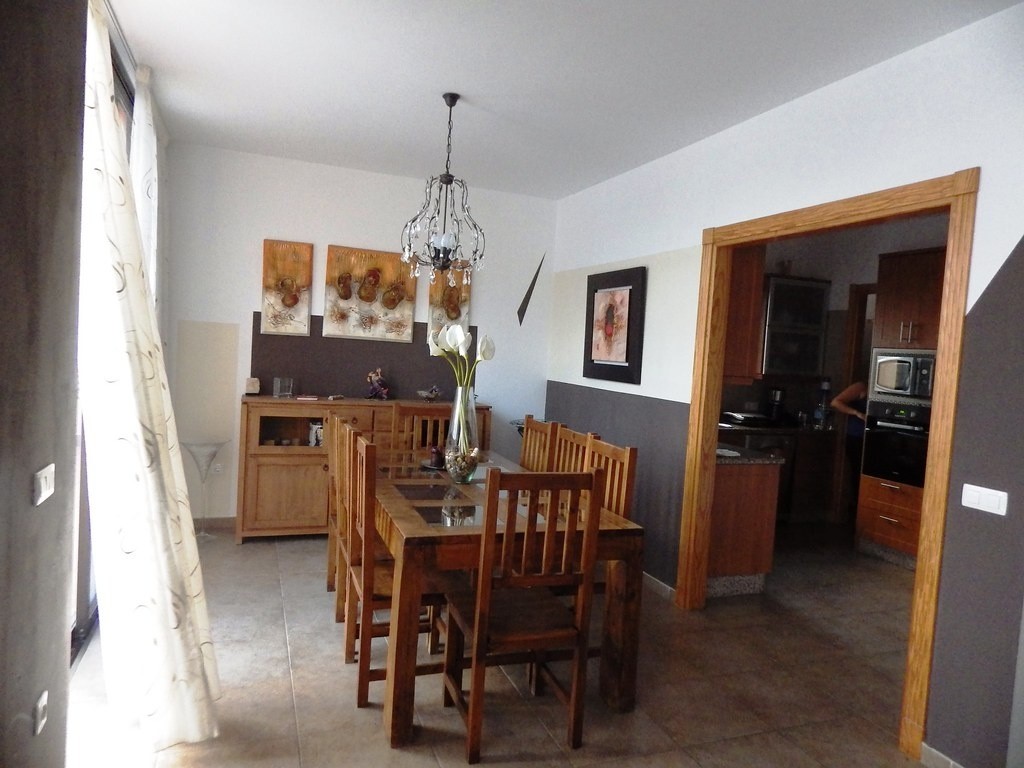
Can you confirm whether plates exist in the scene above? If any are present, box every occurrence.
[421,459,446,470]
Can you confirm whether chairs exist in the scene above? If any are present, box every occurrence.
[323,410,638,765]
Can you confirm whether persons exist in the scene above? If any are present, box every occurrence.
[828,378,867,488]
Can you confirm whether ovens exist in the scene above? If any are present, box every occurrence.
[861,401,931,489]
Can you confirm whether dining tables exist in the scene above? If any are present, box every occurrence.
[372,449,644,748]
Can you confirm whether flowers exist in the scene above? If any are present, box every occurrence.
[422,324,496,455]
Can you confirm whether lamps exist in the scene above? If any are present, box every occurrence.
[400,92,486,289]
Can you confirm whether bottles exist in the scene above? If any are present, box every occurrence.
[811,378,835,432]
[430,446,443,467]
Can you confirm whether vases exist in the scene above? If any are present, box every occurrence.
[445,386,479,484]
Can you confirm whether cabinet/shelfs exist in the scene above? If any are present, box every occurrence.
[706,433,836,598]
[854,474,922,571]
[235,393,491,545]
[874,246,946,349]
[723,243,766,386]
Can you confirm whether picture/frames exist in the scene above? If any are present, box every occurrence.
[582,265,648,384]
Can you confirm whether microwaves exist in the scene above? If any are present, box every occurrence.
[867,347,936,407]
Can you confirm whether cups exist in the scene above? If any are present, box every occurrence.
[245,378,260,396]
[272,377,294,399]
